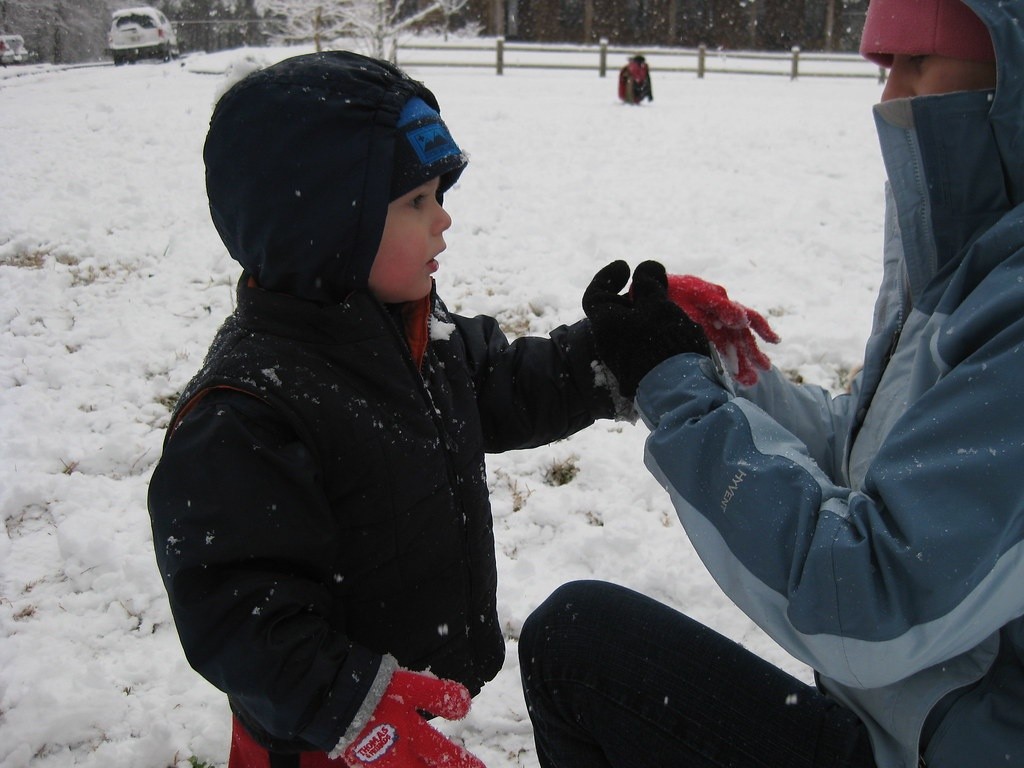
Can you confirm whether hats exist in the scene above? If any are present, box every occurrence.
[387,93,468,201]
[860,0,996,68]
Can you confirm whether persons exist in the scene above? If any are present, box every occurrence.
[520,0,1021,767]
[147,53,781,766]
[618,55,653,105]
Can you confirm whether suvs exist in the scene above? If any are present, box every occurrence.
[107,4,180,64]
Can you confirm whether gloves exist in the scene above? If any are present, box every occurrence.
[630,274,782,387]
[581,260,711,400]
[342,667,487,768]
